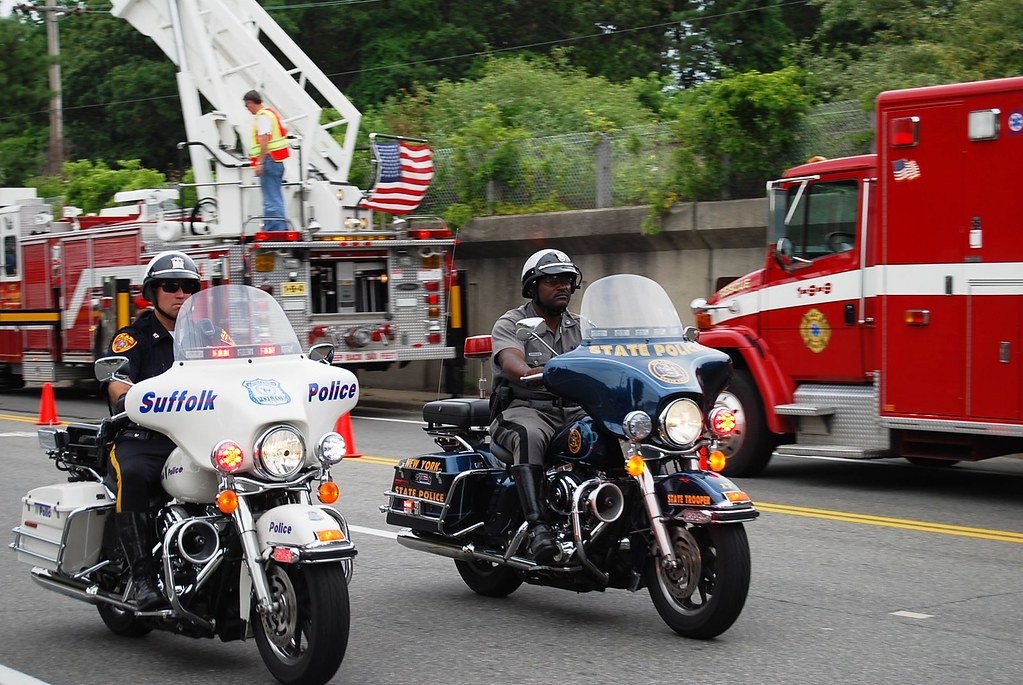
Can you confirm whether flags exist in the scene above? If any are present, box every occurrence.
[359,140,437,214]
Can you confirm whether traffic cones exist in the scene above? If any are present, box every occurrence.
[333,410,361,460]
[33,382,66,427]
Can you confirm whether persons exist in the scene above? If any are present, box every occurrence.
[488,244,599,566]
[243,90,288,232]
[105,248,242,611]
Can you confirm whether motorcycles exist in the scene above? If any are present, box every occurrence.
[376,272,761,640]
[10,280,358,685]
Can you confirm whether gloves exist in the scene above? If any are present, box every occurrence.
[112,392,147,429]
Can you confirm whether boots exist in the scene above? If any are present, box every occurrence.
[509,462,562,562]
[115,509,167,612]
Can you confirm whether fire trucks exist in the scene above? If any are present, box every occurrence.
[0,0,458,398]
[680,80,1023,475]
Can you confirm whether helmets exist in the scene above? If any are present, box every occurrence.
[521,247,579,299]
[142,250,203,303]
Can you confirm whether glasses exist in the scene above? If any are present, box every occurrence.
[542,276,574,283]
[245,101,253,107]
[153,280,196,293]
